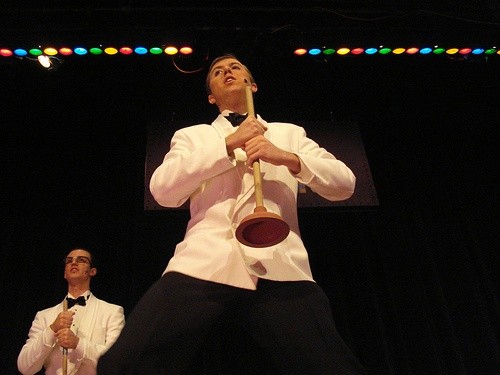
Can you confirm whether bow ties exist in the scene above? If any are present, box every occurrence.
[66,296,86,308]
[224,112,257,127]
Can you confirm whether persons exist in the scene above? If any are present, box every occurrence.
[97,54,366,375]
[17,248,126,375]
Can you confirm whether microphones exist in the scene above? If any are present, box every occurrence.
[244,78,255,96]
[86,270,93,274]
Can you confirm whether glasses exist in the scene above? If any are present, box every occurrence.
[63,256,91,264]
